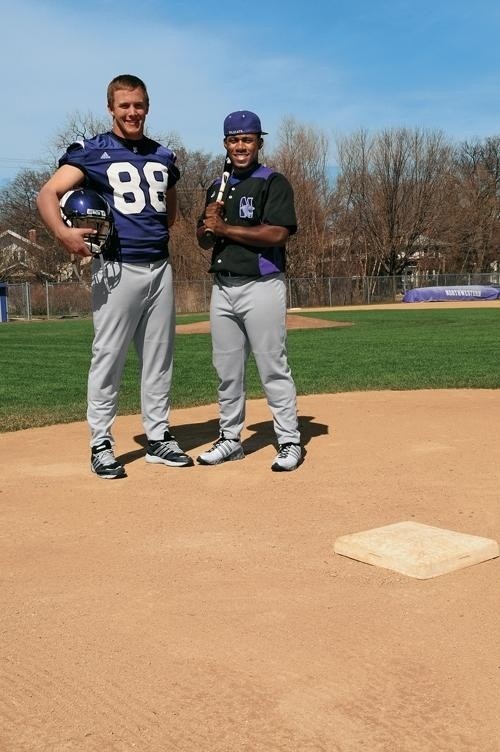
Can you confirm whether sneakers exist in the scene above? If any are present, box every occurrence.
[196,438,245,464]
[91,440,126,478]
[145,431,193,467]
[271,442,303,471]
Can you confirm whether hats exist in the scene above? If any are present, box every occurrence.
[224,111,268,135]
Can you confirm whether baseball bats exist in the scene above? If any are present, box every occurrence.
[205,153,233,238]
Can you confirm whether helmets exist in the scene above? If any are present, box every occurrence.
[59,188,115,254]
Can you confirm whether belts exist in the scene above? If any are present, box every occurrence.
[221,270,240,277]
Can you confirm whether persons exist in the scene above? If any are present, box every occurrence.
[197,110,302,471]
[37,74,194,479]
